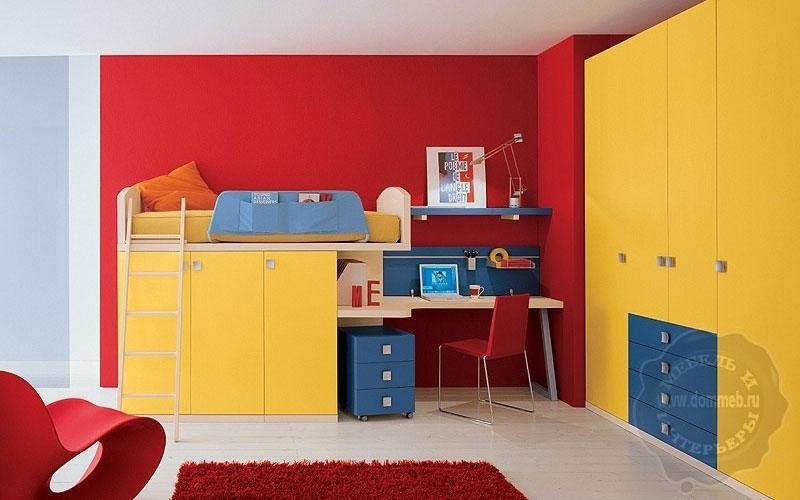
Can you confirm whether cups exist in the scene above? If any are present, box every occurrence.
[469,285,484,299]
[467,257,476,270]
[509,198,520,208]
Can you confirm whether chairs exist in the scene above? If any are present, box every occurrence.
[436,292,537,422]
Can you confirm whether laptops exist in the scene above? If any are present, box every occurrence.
[419,264,472,302]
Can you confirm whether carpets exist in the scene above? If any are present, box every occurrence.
[166,460,527,500]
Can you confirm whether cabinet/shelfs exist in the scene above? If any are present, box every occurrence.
[583,0,800,499]
[116,252,342,416]
[344,328,415,422]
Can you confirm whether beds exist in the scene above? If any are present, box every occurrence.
[115,184,415,443]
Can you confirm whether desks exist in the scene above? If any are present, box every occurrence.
[338,292,565,415]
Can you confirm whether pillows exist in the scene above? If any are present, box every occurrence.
[130,162,218,213]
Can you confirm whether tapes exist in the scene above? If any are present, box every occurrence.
[490,248,509,267]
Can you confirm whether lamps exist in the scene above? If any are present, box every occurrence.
[466,129,528,209]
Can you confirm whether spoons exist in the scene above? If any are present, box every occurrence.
[462,248,479,258]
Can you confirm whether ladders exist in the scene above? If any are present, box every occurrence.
[118,198,186,442]
